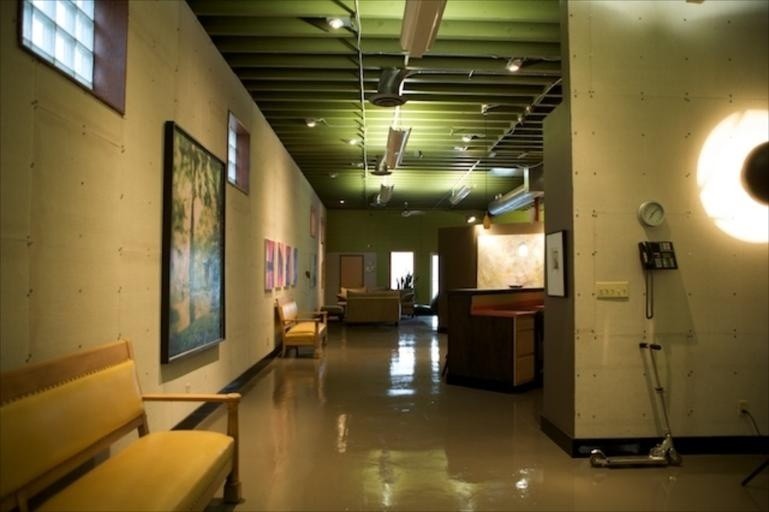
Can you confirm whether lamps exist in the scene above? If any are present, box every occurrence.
[398,0,448,60]
[372,127,543,231]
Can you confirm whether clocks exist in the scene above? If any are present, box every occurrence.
[639,199,667,229]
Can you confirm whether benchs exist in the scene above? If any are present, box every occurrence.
[1,339,245,510]
[273,295,329,360]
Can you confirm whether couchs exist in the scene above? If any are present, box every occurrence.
[322,283,416,329]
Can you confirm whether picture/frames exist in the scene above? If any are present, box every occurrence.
[157,119,229,366]
[543,229,570,301]
[263,239,300,292]
[338,253,365,294]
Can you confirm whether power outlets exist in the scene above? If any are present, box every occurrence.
[738,399,750,418]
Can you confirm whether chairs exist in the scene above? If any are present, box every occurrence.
[411,291,439,318]
